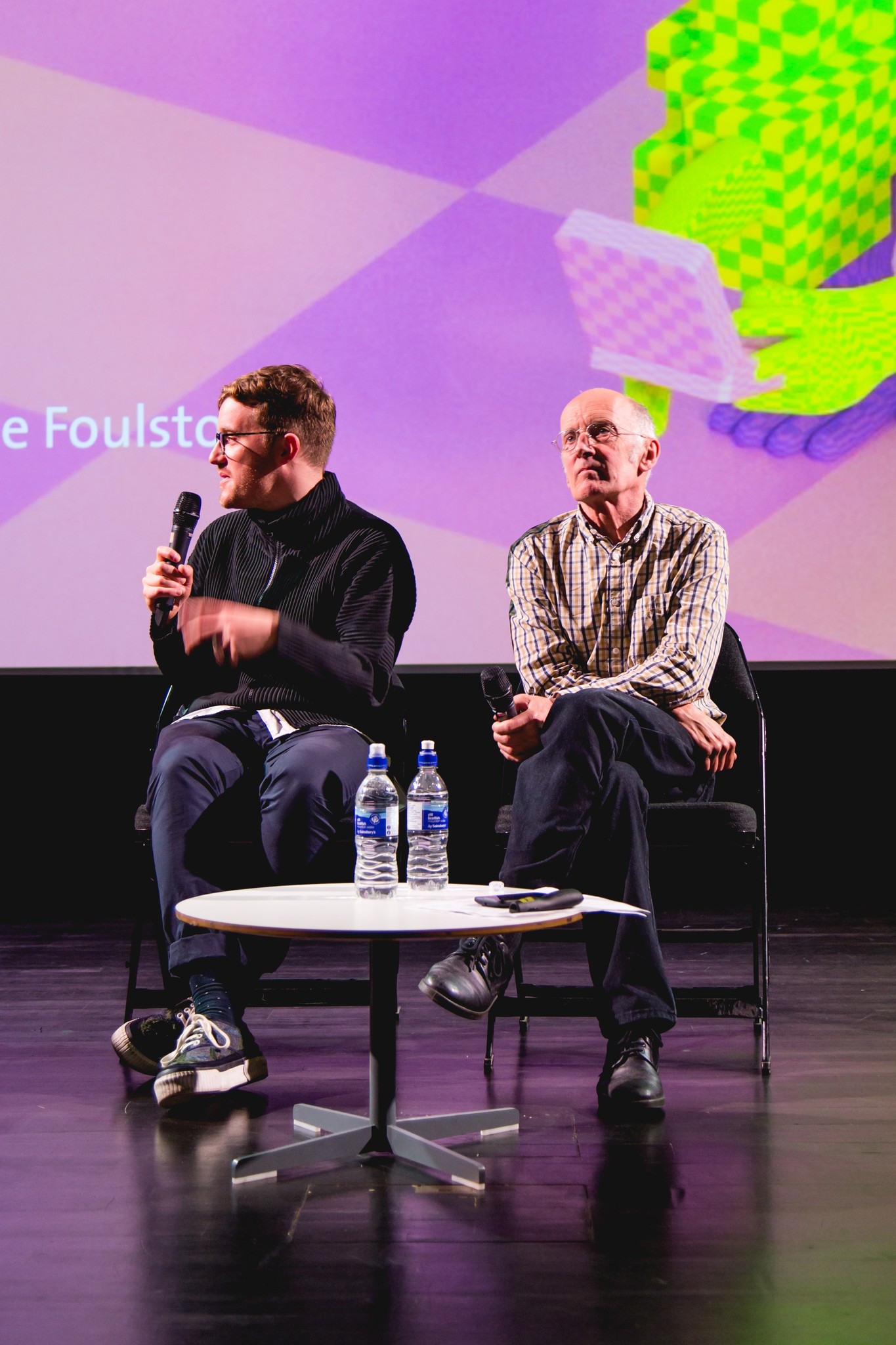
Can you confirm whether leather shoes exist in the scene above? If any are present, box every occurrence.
[419,932,515,1018]
[597,1027,665,1113]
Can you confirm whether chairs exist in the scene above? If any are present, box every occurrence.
[482,621,771,1081]
[117,684,410,1069]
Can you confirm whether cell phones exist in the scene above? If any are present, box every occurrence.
[475,892,549,908]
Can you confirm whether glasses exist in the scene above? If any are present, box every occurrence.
[550,420,653,451]
[216,431,296,456]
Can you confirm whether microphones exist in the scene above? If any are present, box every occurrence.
[480,665,517,723]
[155,491,202,610]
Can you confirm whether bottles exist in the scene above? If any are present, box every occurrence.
[406,740,450,891]
[353,743,399,901]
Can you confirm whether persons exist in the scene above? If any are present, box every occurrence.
[415,387,738,1111]
[113,365,416,1109]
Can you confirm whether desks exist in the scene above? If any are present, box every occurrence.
[175,883,582,1193]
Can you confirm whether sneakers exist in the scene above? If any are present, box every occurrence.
[155,1003,269,1107]
[110,996,199,1078]
